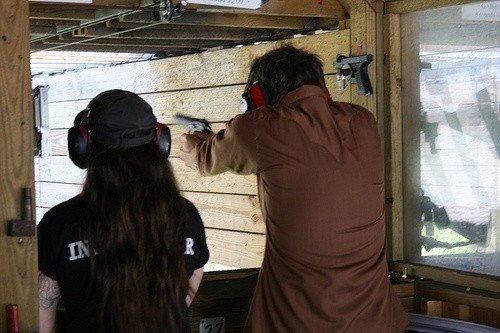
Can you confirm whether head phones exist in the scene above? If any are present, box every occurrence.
[240,63,332,114]
[68,89,171,169]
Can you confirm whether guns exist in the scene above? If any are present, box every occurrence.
[173,113,215,133]
[419,189,492,252]
[336,53,374,97]
[420,112,438,154]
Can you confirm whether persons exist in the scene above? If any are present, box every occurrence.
[39,89,210,333]
[179,46,410,332]
